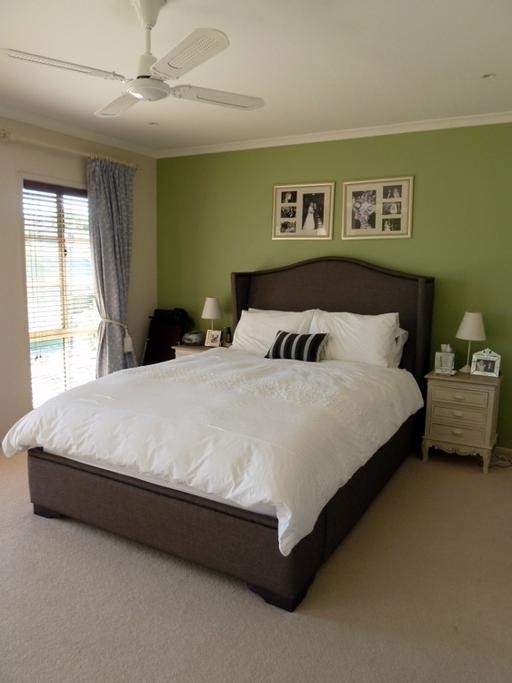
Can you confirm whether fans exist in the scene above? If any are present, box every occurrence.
[0,0,266,119]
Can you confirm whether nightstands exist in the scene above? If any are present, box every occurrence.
[419,370,504,475]
[171,345,207,358]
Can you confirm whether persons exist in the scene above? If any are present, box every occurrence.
[303,201,321,229]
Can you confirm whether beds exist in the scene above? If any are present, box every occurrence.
[2,255,435,614]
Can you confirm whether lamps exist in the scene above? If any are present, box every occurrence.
[201,297,223,329]
[456,312,487,373]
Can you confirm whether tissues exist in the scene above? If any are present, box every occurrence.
[435,344,457,375]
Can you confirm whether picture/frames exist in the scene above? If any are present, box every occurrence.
[272,181,335,240]
[471,348,501,377]
[205,330,221,348]
[340,175,414,241]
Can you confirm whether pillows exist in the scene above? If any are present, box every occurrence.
[229,310,314,357]
[307,309,400,368]
[264,331,331,361]
